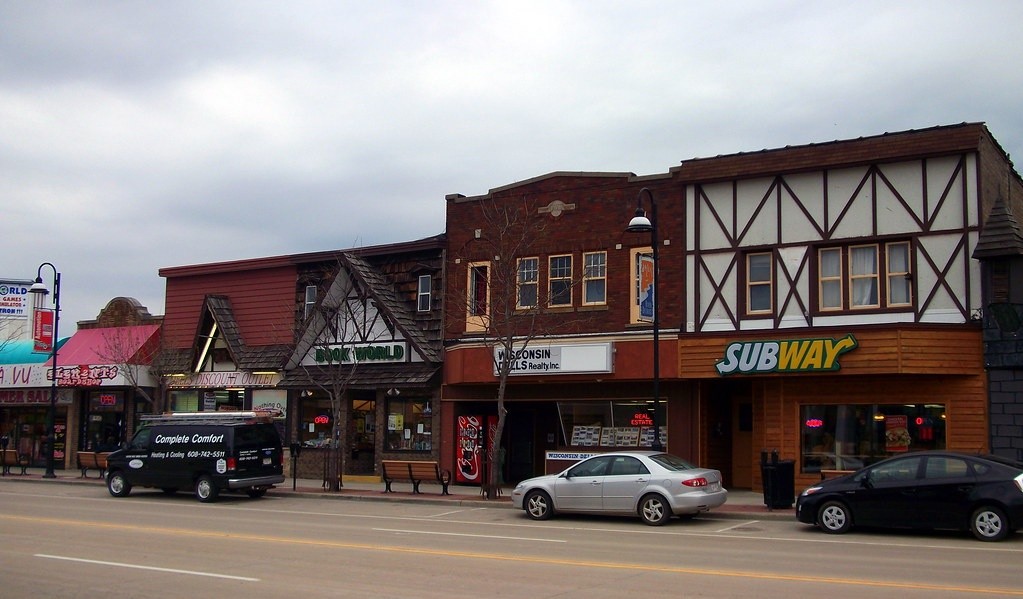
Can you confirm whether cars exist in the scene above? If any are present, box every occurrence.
[795,450,1023,542]
[510,451,728,526]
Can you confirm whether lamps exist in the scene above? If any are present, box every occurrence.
[387,387,401,396]
[301,390,312,398]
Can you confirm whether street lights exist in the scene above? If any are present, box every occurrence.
[624,187,662,448]
[26,262,62,478]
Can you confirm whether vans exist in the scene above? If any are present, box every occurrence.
[102,409,286,503]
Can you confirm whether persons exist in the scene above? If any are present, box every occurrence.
[389,433,407,449]
[810,430,834,463]
[498,444,507,485]
[102,431,111,443]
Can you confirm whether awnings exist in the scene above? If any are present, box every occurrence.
[0,364,161,388]
[276,362,442,390]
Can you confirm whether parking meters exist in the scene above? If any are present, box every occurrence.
[761,448,779,509]
[1,436,10,476]
[290,441,301,489]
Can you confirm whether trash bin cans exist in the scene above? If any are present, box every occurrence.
[761,459,795,509]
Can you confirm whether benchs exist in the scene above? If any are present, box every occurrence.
[77,451,111,479]
[382,460,452,495]
[820,470,856,479]
[0,449,29,476]
